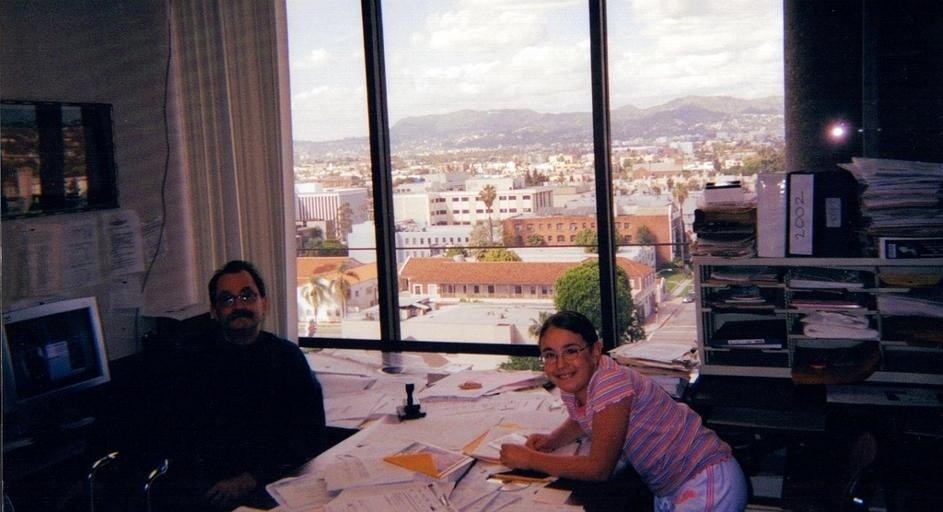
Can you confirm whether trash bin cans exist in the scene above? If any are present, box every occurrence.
[781,474,865,512]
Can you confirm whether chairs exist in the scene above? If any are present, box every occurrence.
[85,452,120,511]
[143,458,177,511]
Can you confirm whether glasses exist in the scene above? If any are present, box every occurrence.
[538,344,592,361]
[214,290,259,307]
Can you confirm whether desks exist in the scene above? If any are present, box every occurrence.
[270,351,700,511]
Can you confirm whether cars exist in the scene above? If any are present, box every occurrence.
[682,293,695,303]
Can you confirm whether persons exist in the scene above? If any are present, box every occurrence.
[171,260,328,511]
[499,311,749,512]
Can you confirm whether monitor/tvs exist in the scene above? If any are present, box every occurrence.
[0,296,111,405]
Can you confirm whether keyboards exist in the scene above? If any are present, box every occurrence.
[707,407,827,431]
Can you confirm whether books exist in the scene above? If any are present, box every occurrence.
[607,339,699,401]
[690,159,943,406]
[382,440,473,481]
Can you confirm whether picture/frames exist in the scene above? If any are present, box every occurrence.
[0,99,121,221]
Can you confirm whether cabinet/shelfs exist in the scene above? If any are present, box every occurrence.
[692,255,943,384]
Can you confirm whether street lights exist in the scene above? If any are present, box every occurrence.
[654,269,673,324]
